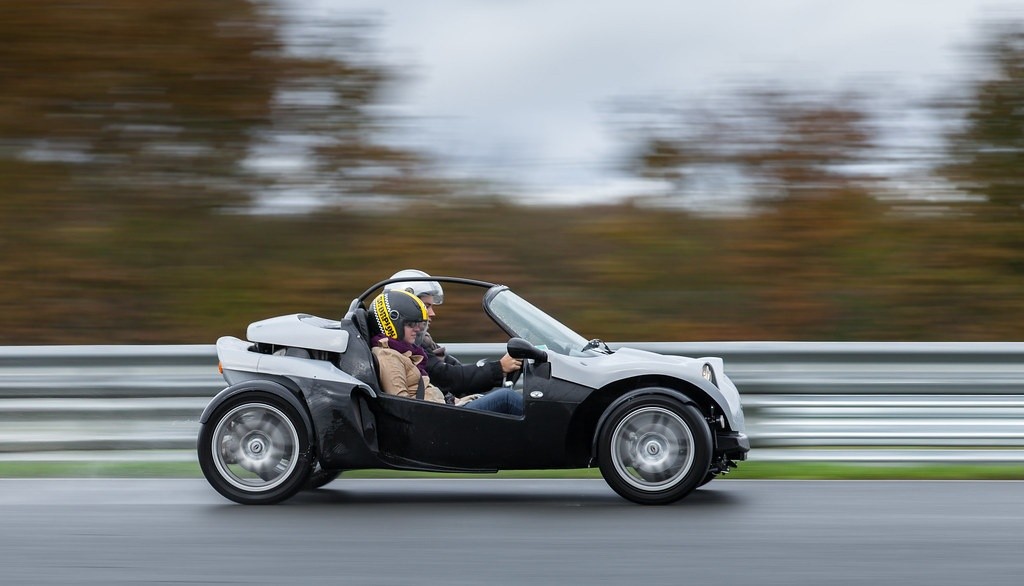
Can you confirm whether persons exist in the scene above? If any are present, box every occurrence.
[368,289,524,418]
[383,268,524,399]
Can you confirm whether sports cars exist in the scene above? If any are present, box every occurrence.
[194,276,750,507]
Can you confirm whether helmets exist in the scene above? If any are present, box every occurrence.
[367,290,429,347]
[383,269,444,305]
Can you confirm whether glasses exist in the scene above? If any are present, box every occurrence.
[404,322,419,328]
